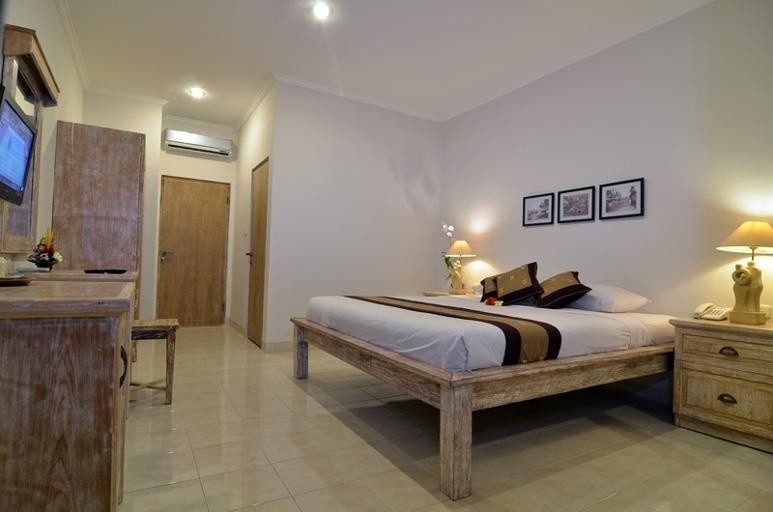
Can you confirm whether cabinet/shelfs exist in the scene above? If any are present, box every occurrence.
[14,265,139,421]
[0,282,136,510]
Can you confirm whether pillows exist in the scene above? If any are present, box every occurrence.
[477,260,653,315]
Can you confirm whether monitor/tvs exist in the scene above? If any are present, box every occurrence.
[0,84,38,208]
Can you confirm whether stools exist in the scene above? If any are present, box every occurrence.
[128,315,180,406]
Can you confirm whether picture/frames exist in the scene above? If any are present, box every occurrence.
[520,191,555,227]
[556,185,596,223]
[596,176,644,220]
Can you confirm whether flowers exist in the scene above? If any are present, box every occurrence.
[438,220,456,280]
[22,224,65,270]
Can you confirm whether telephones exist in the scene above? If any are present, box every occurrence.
[692,302,734,320]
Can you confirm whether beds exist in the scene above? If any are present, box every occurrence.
[283,287,678,503]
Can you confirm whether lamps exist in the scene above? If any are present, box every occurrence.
[440,238,477,295]
[711,219,773,328]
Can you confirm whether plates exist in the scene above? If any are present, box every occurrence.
[0,277,32,286]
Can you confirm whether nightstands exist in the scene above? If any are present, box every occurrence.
[665,303,771,456]
[419,288,479,299]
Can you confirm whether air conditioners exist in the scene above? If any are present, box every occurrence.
[162,127,233,164]
